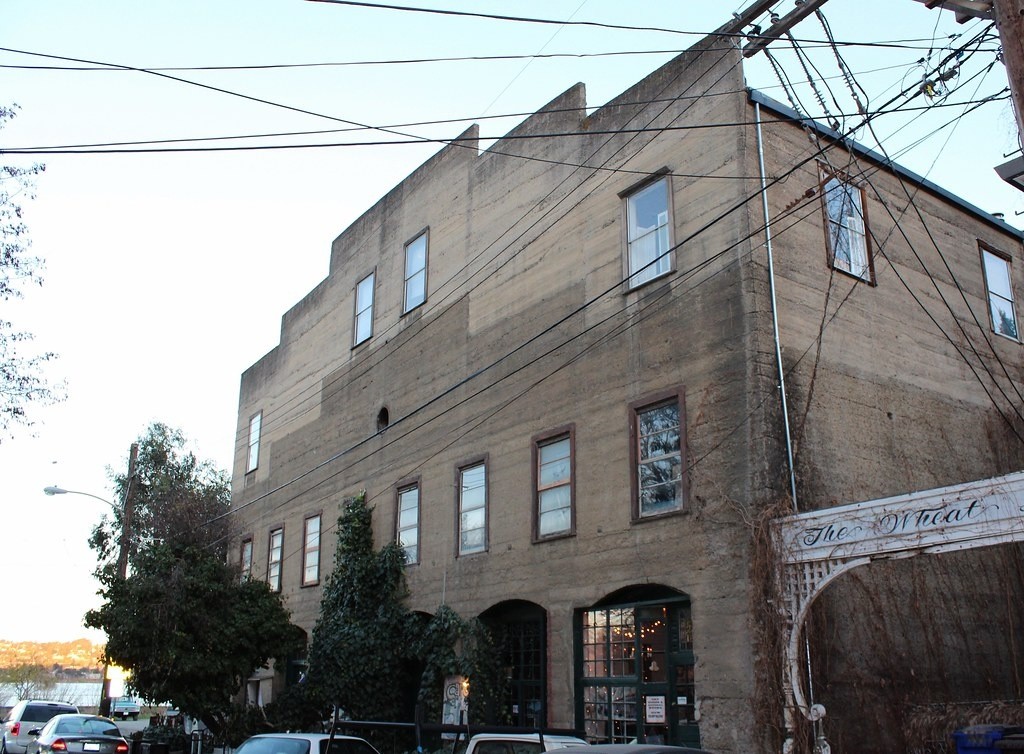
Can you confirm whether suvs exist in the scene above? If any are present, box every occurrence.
[0,699,80,754]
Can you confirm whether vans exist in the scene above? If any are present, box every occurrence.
[164,702,180,720]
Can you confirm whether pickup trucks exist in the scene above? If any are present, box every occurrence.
[111,696,140,721]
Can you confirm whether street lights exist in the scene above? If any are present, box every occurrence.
[43,486,129,716]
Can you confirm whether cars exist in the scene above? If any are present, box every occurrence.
[466,734,589,754]
[25,714,128,754]
[540,744,714,753]
[235,734,378,753]
